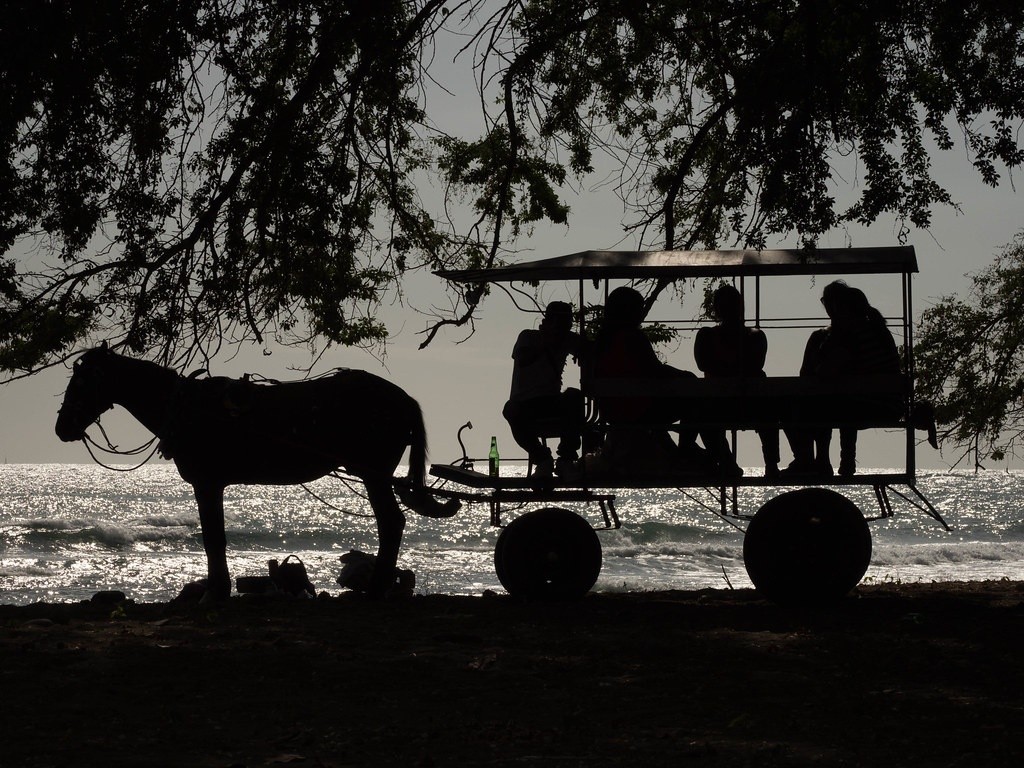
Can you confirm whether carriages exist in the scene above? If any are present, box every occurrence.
[56,246,952,607]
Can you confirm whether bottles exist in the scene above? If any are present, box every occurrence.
[489,437,499,476]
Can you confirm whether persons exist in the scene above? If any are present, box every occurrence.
[694,286,780,478]
[591,287,700,476]
[502,301,592,480]
[783,283,906,474]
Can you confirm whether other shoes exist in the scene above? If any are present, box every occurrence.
[716,455,745,485]
[762,454,859,479]
[529,457,584,486]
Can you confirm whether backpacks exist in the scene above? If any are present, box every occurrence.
[278,554,313,605]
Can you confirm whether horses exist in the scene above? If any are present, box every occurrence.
[56,340,460,608]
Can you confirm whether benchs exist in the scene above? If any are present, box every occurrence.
[694,370,914,461]
